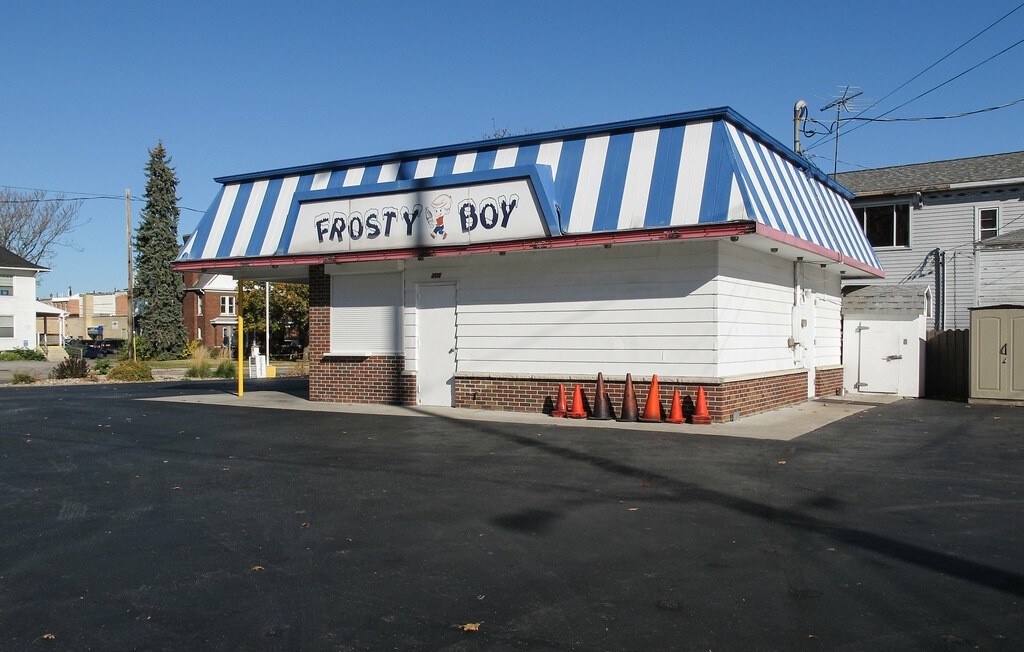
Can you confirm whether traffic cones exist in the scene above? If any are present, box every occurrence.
[640,374,664,423]
[614,373,640,422]
[692,386,712,425]
[666,388,687,423]
[551,384,569,418]
[588,374,615,420]
[568,384,588,419]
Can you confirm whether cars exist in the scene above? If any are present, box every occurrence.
[82,346,107,359]
[283,340,295,347]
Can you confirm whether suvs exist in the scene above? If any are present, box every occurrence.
[65,338,127,353]
